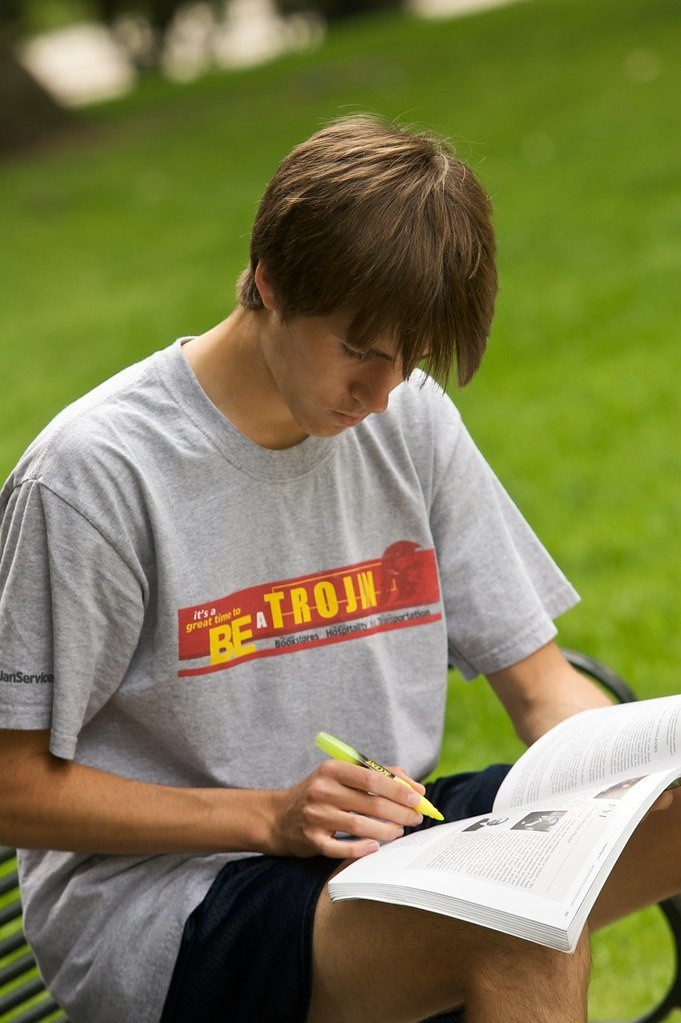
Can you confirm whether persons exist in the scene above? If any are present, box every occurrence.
[0,107,680,1023]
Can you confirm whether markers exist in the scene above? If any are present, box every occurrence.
[315,731,446,822]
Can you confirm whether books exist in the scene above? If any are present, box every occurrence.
[325,689,680,955]
